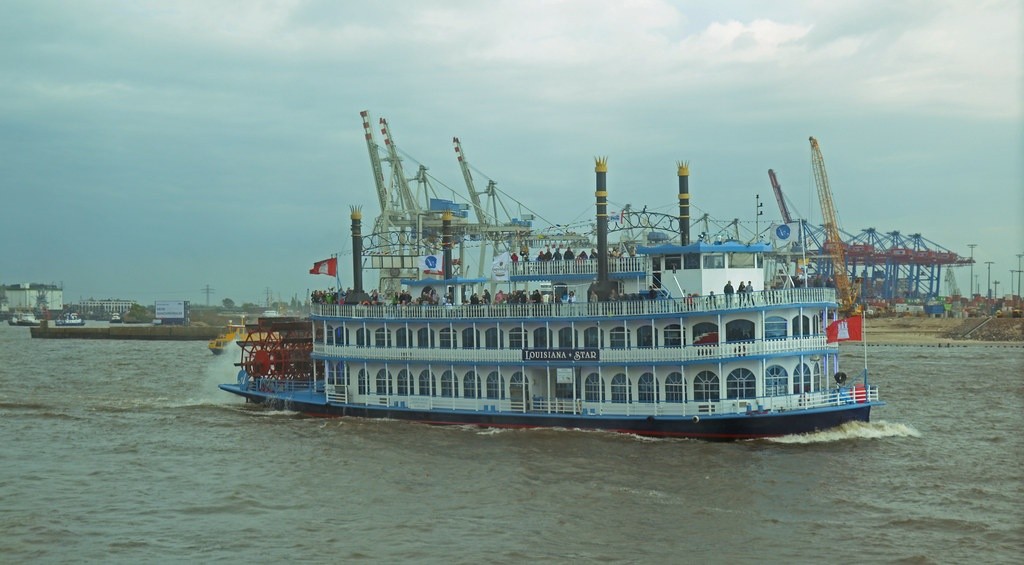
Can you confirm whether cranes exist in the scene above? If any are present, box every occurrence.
[346,105,863,317]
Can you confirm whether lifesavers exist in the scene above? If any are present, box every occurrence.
[833,371,848,383]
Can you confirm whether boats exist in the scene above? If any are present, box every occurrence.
[54,314,86,326]
[110,312,123,323]
[8,310,41,326]
[218,113,888,442]
[207,315,276,356]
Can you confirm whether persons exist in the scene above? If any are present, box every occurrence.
[509,245,637,275]
[310,285,380,316]
[464,289,577,316]
[681,280,756,310]
[391,289,454,316]
[793,272,836,302]
[589,286,658,313]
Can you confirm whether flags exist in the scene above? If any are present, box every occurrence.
[418,253,443,272]
[825,314,862,344]
[309,258,336,277]
[606,208,625,224]
[769,221,799,248]
